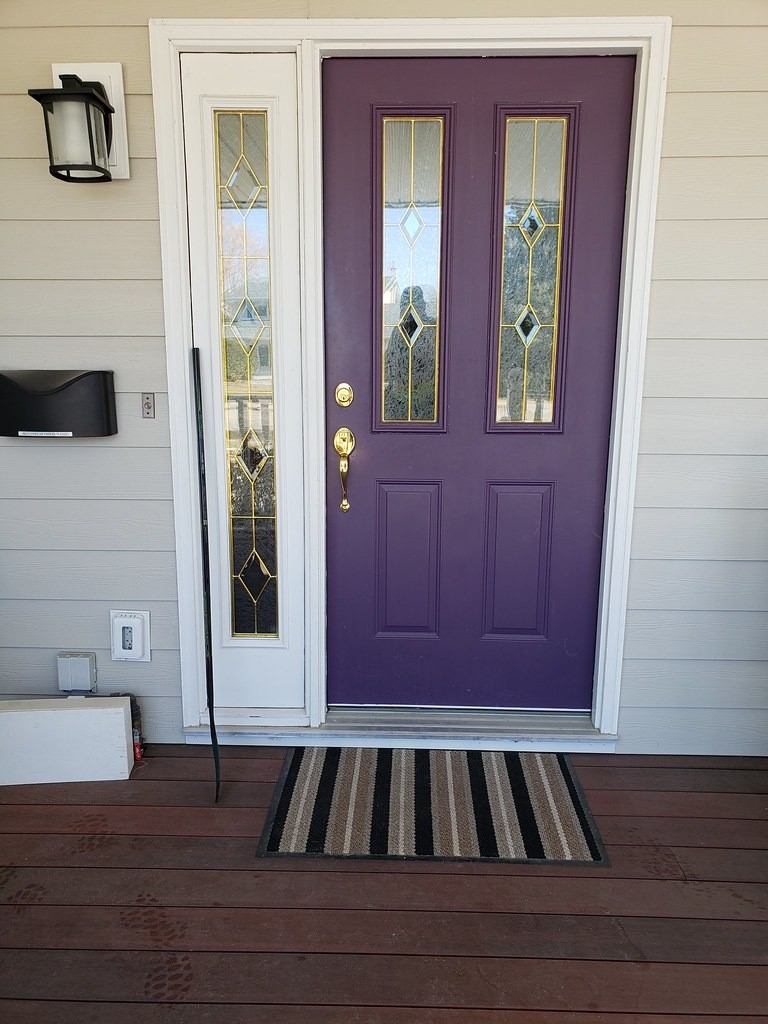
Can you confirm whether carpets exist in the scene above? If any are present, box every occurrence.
[256,746,611,869]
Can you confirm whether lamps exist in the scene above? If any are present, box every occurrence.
[28,75,115,183]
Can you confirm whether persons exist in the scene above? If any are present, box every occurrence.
[506,363,530,420]
[384,286,434,421]
